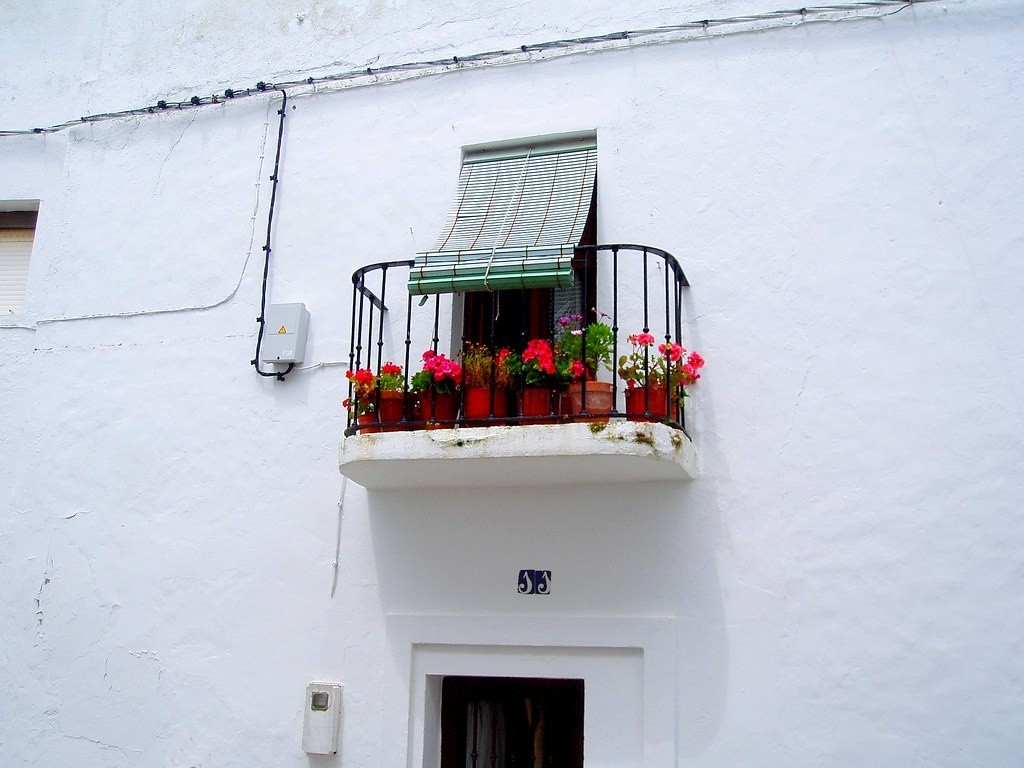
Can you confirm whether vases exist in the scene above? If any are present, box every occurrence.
[668,390,681,422]
[623,387,668,424]
[564,380,615,424]
[412,391,460,430]
[512,388,561,427]
[356,414,383,435]
[459,385,511,427]
[374,389,406,430]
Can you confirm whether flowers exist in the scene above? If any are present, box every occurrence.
[660,341,702,394]
[457,338,494,385]
[512,338,556,382]
[491,347,515,385]
[341,367,378,417]
[619,331,663,390]
[556,306,616,384]
[410,348,462,393]
[376,362,406,391]
